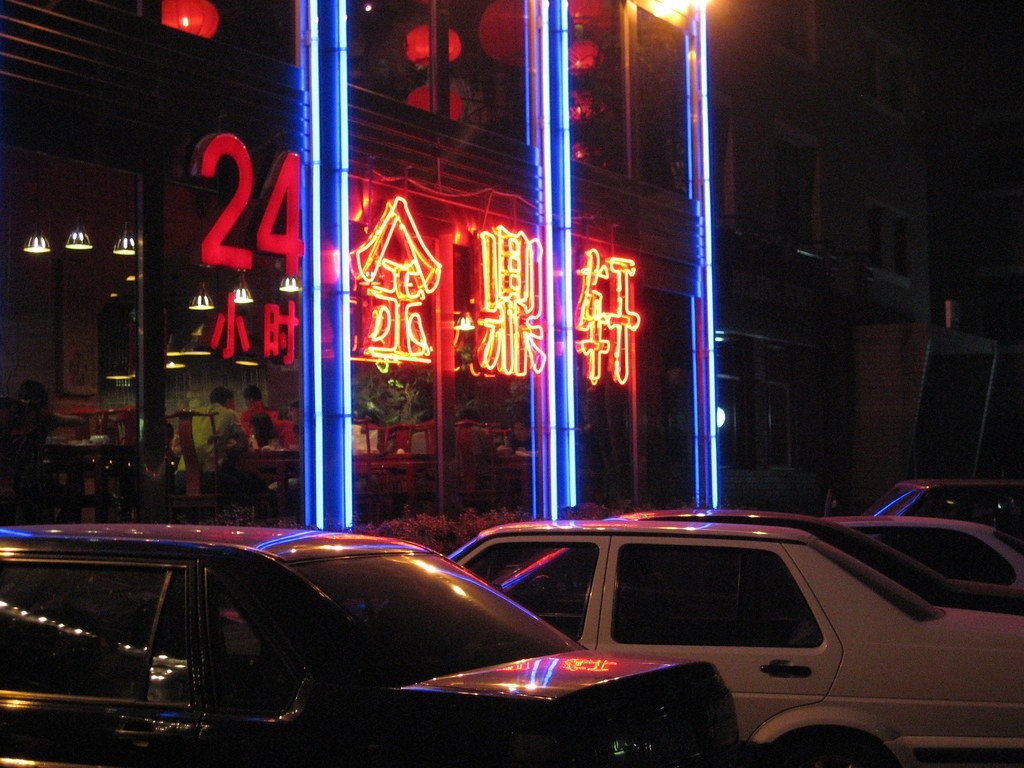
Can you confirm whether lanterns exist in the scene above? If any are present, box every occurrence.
[568,0,612,33]
[570,91,607,125]
[403,24,462,69]
[407,83,462,121]
[572,142,609,169]
[479,0,524,65]
[569,40,606,77]
[160,0,220,40]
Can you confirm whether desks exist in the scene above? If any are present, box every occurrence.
[354,449,530,524]
[234,449,304,526]
[40,442,140,527]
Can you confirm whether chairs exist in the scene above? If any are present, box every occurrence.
[1,407,530,525]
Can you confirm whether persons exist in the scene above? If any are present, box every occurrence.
[0,379,86,525]
[455,409,494,474]
[509,415,530,454]
[165,385,301,525]
[410,410,435,456]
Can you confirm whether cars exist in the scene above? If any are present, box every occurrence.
[446,477,1024,768]
[0,516,744,768]
